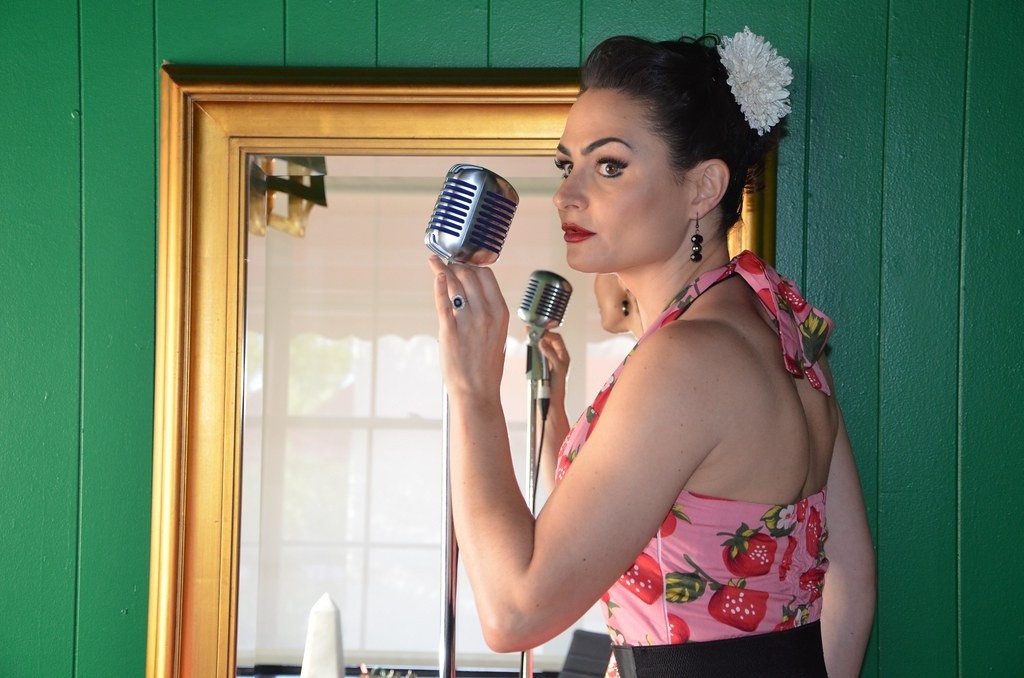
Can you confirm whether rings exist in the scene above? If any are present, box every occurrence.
[450,295,470,310]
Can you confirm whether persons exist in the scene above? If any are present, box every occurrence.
[429,35,877,678]
[527,272,645,678]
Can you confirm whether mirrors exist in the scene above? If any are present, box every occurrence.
[143,61,780,678]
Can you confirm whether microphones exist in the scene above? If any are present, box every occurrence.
[517,270,574,418]
[424,163,520,268]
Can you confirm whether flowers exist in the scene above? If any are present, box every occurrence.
[717,25,794,137]
